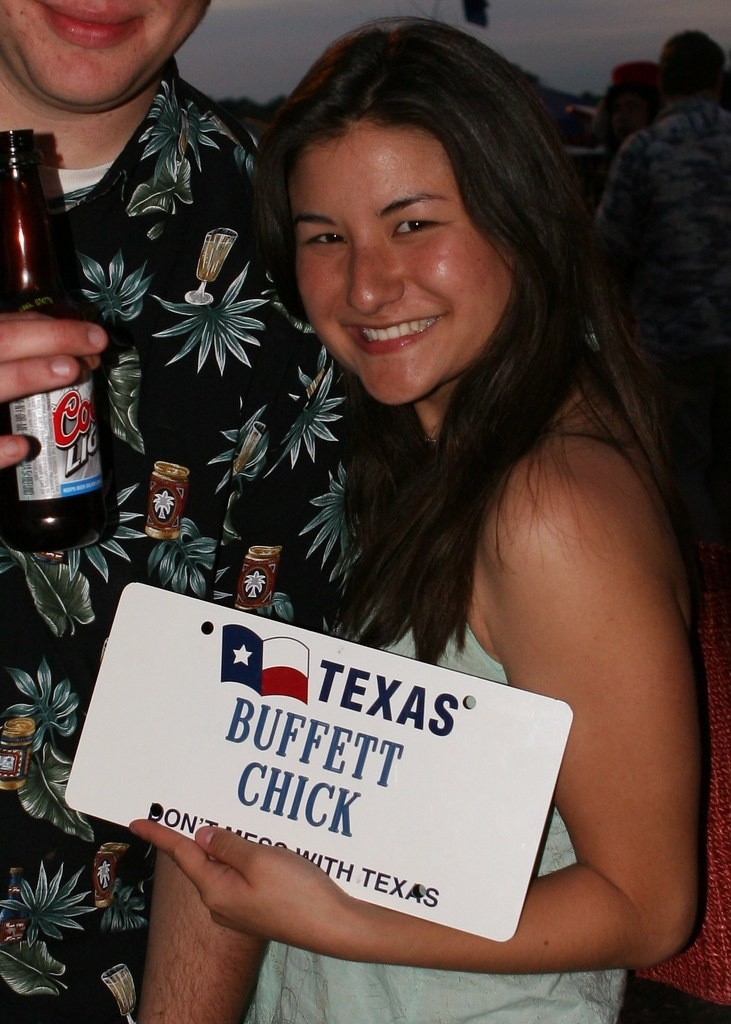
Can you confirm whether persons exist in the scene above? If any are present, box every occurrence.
[595,61,663,163]
[0,0,432,1024]
[132,16,703,1024]
[592,31,731,370]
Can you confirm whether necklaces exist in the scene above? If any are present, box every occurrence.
[47,183,96,208]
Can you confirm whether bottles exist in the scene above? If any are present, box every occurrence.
[1,126,112,550]
